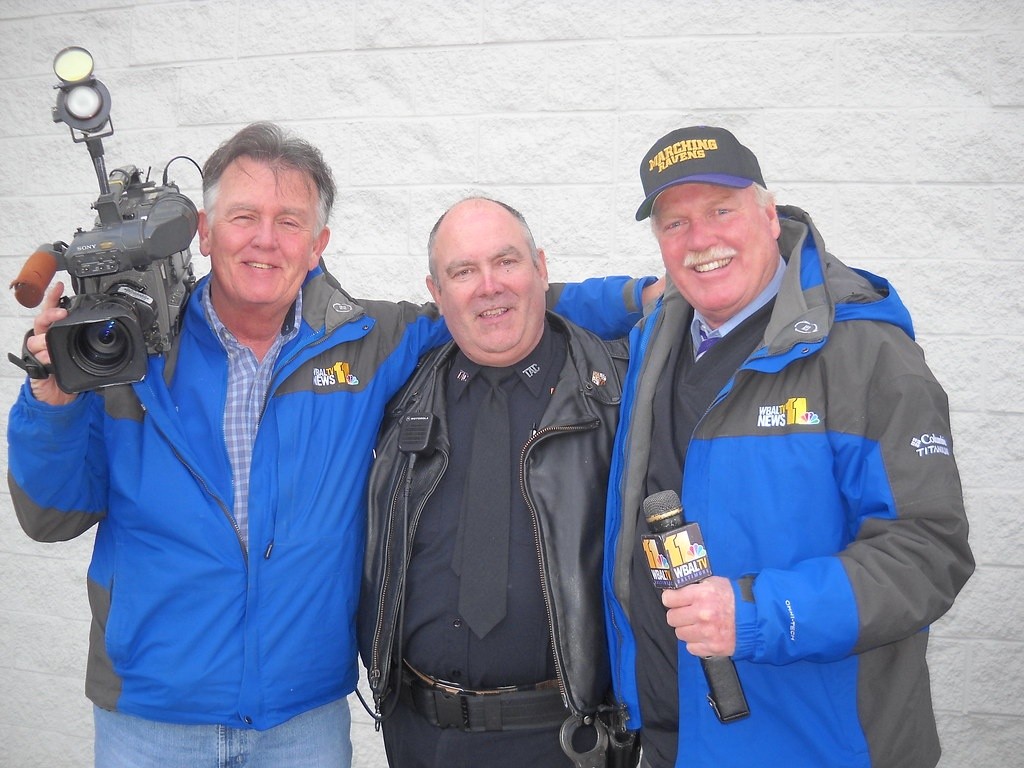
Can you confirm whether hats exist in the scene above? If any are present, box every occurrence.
[636,126,767,220]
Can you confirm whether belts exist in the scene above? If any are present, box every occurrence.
[400,684,572,733]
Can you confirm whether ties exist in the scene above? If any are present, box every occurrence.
[457,365,521,640]
[695,323,721,364]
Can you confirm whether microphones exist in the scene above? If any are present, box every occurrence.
[640,490,751,722]
[8,250,57,310]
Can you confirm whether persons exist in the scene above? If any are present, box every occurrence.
[358,198,633,768]
[6,122,666,768]
[604,125,976,768]
